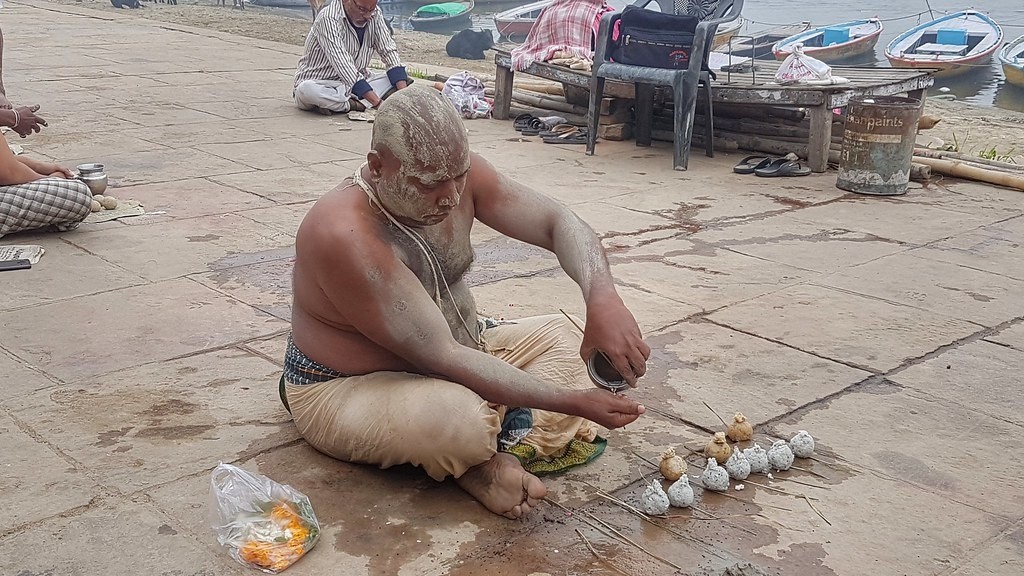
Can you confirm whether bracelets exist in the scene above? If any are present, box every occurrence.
[375,100,382,107]
[8,109,20,128]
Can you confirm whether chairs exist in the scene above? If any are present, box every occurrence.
[585,0,744,172]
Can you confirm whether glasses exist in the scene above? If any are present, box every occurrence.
[354,0,378,14]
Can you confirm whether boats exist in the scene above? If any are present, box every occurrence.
[885,9,1004,81]
[771,16,885,63]
[997,35,1024,89]
[713,21,812,60]
[713,12,745,54]
[492,0,552,38]
[407,0,476,28]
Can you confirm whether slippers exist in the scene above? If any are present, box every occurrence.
[522,117,553,136]
[734,155,773,174]
[513,113,532,131]
[543,131,599,143]
[539,124,588,138]
[755,158,812,177]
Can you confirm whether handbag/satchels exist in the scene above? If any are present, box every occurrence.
[209,462,321,576]
[774,42,832,85]
[612,4,717,81]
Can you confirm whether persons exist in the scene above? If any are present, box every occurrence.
[0,30,93,240]
[293,0,408,115]
[283,85,651,520]
